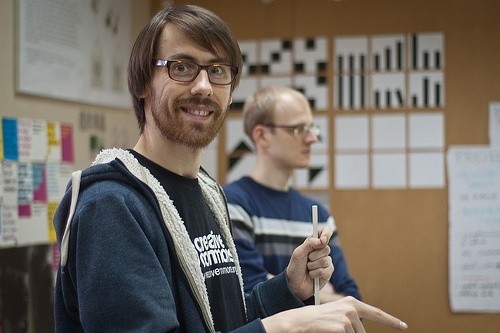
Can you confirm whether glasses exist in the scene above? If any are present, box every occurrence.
[153,59,239,85]
[260,122,320,137]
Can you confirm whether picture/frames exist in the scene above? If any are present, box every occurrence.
[13,1,140,114]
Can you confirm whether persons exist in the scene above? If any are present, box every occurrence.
[43,0,408,333]
[214,86,364,303]
